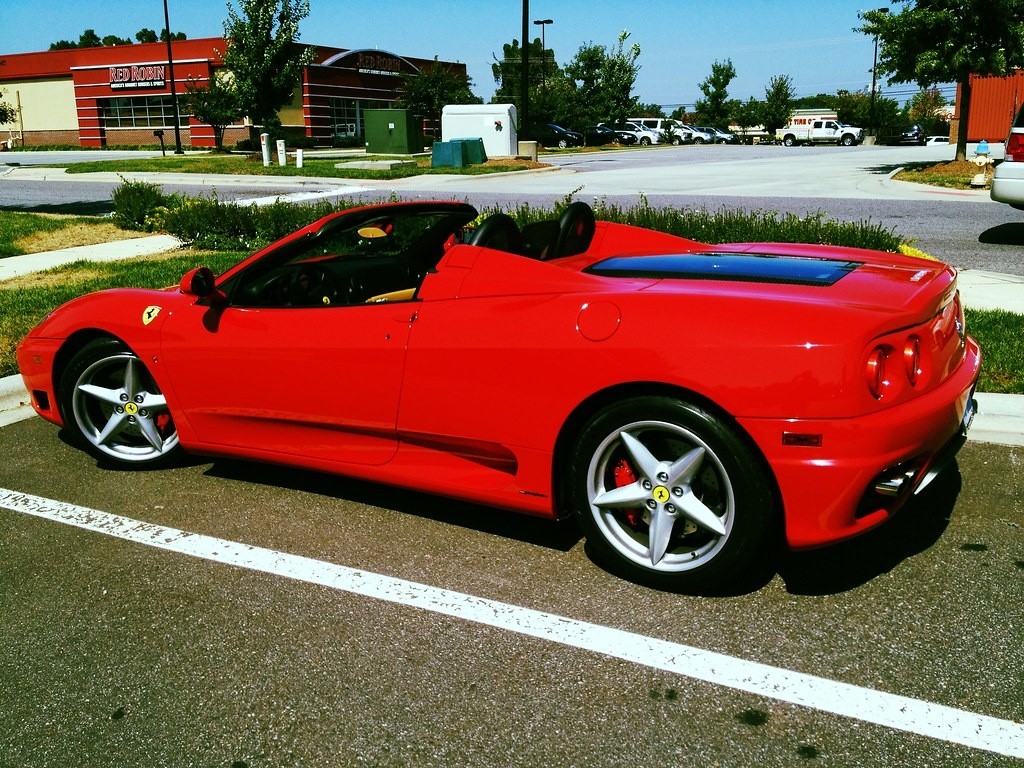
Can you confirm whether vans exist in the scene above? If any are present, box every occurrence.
[595,121,662,146]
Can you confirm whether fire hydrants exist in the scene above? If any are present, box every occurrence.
[970,139,995,188]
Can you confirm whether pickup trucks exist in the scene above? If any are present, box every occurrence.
[775,118,865,147]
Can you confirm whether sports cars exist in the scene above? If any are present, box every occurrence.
[15,201,983,595]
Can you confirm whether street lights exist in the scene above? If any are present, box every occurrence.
[869,8,889,137]
[534,19,553,90]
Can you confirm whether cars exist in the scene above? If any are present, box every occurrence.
[575,125,618,146]
[899,123,928,147]
[537,123,585,150]
[991,102,1024,210]
[925,135,949,146]
[616,117,741,145]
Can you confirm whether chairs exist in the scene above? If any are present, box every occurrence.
[467,201,596,261]
